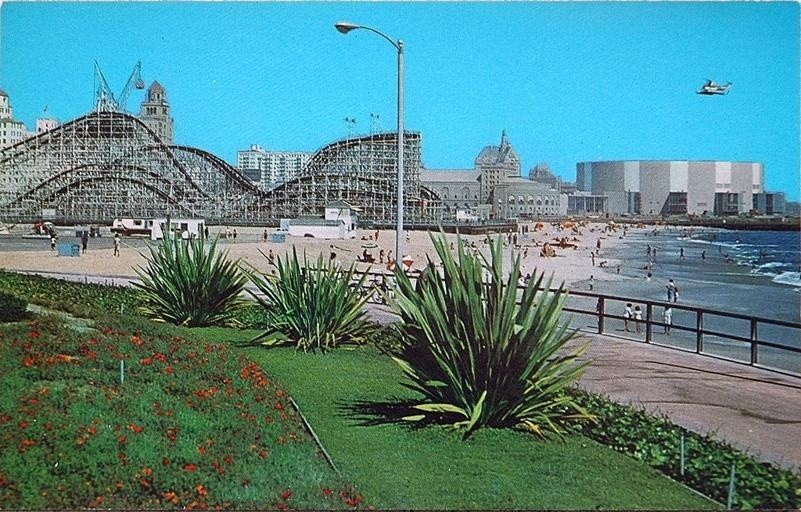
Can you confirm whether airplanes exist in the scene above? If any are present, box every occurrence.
[696,78,734,96]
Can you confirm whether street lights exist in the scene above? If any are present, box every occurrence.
[336,22,406,302]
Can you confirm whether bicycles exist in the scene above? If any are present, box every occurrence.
[345,276,387,303]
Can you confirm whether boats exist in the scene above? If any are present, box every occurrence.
[22,233,58,241]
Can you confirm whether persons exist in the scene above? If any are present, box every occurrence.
[50,234,57,250]
[112,234,121,257]
[166,210,722,334]
[81,234,89,254]
[35,222,43,234]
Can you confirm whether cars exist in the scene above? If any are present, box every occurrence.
[273,228,290,235]
